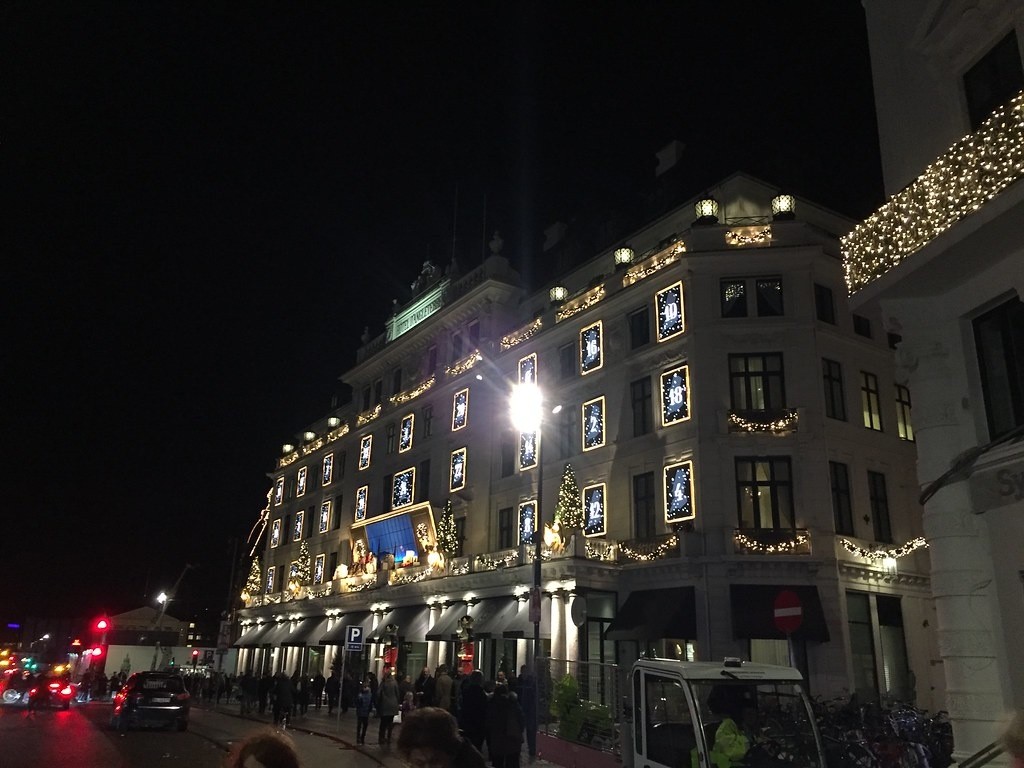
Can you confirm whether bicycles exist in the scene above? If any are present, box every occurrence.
[754,693,947,768]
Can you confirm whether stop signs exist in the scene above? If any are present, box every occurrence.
[772,591,804,634]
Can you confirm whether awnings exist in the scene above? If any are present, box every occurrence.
[450,600,489,640]
[365,605,429,643]
[503,594,551,640]
[475,599,519,640]
[280,616,327,647]
[232,624,259,649]
[728,583,831,644]
[244,620,291,648]
[319,610,373,646]
[425,601,467,642]
[602,586,697,642]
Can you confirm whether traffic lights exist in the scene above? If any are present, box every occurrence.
[171,657,174,669]
[192,650,199,665]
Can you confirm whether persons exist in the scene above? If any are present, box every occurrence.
[183,671,245,706]
[77,666,126,702]
[324,671,378,714]
[228,726,302,768]
[356,681,374,746]
[25,672,52,720]
[239,668,326,731]
[398,664,544,768]
[376,672,402,744]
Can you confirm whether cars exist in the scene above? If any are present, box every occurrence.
[109,671,190,735]
[0,675,71,711]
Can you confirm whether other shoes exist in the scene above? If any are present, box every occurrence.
[387,738,392,743]
[362,736,364,744]
[357,738,360,743]
[378,739,386,744]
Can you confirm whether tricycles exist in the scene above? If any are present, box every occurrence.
[629,652,825,768]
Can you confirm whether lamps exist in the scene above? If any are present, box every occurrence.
[282,440,294,457]
[455,617,469,656]
[517,589,529,601]
[430,601,455,610]
[612,241,634,270]
[241,615,306,627]
[328,413,341,431]
[374,629,391,645]
[329,611,344,620]
[467,598,481,608]
[550,281,569,305]
[693,191,719,223]
[545,575,577,591]
[868,543,898,570]
[303,427,316,445]
[373,607,393,616]
[771,187,795,220]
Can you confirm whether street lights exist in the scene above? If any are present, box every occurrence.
[511,385,544,763]
